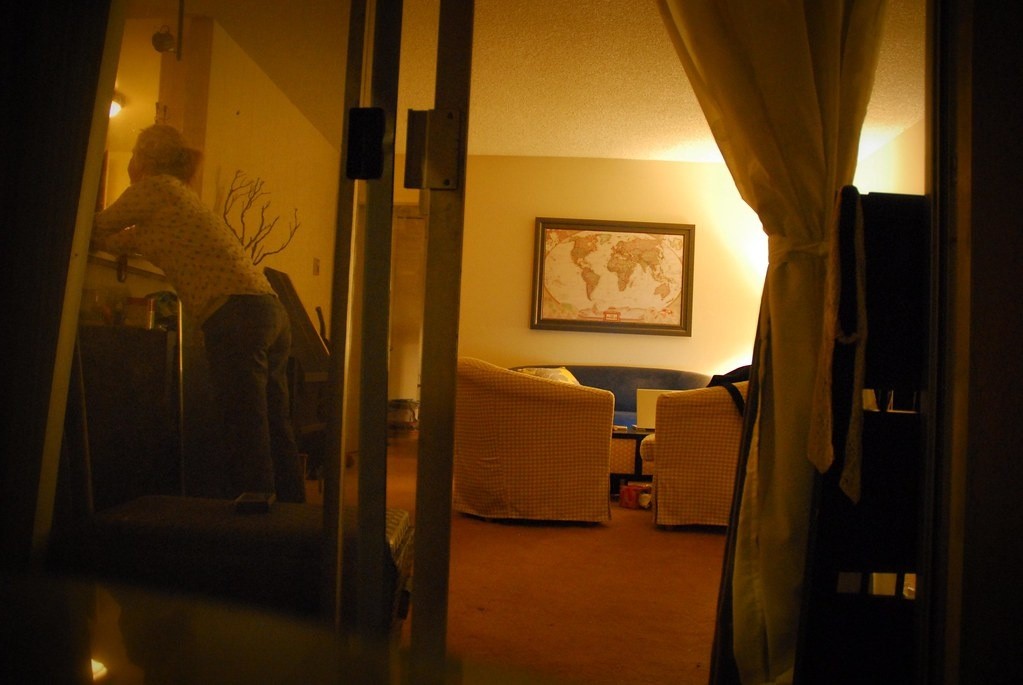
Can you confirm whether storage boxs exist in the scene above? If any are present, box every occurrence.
[618,484,652,509]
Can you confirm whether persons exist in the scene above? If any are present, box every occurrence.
[85,122,310,504]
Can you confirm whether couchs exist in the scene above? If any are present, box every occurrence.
[506,364,713,428]
[639,378,753,530]
[453,356,614,523]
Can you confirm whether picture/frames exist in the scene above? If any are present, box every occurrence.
[531,216,695,339]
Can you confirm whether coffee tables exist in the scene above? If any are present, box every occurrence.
[610,425,654,486]
